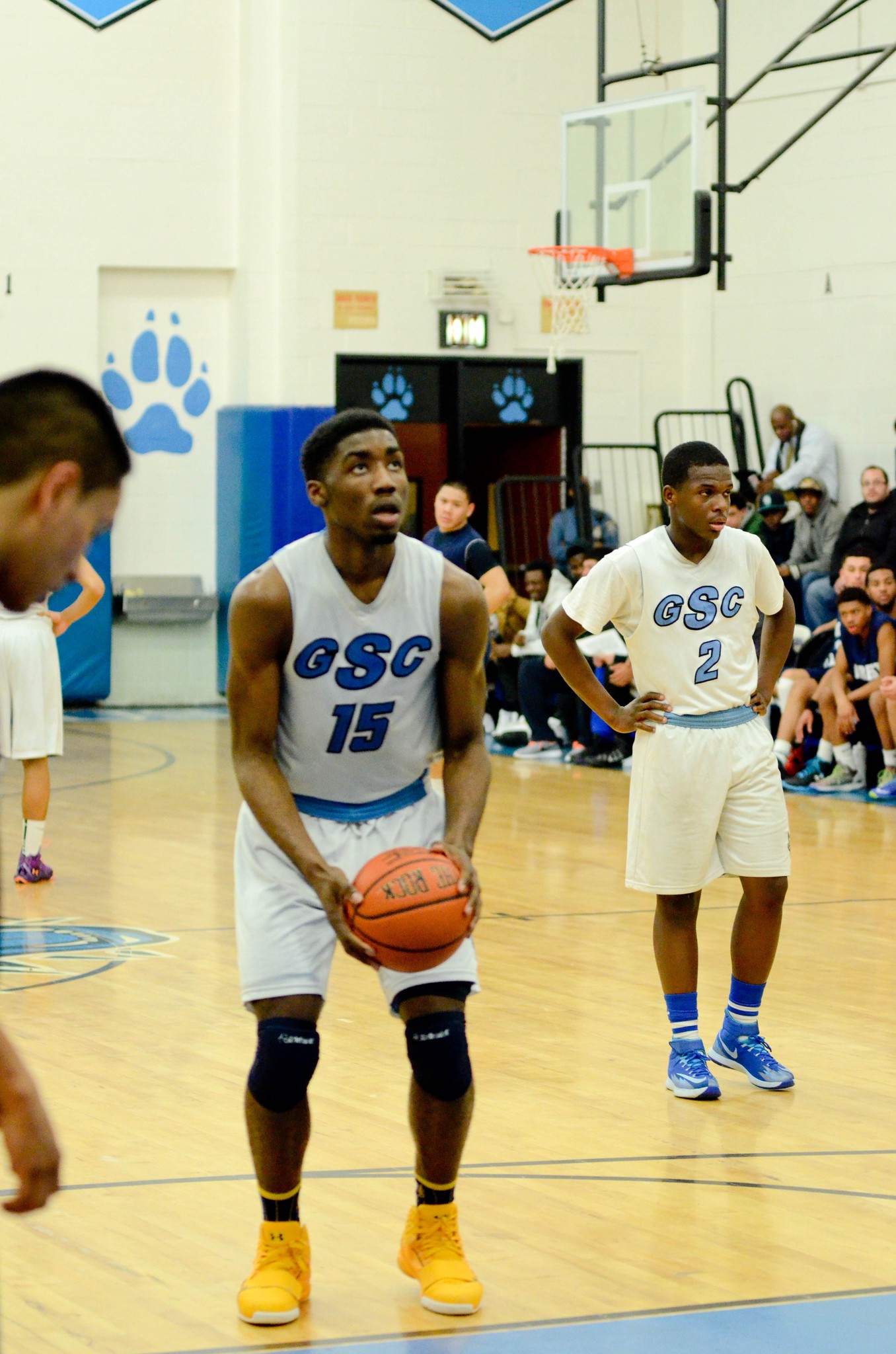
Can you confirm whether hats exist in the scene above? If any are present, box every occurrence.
[796,478,825,501]
[755,489,787,516]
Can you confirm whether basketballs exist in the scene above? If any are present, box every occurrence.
[343,846,471,974]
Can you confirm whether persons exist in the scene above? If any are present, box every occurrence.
[722,404,896,800]
[420,475,638,773]
[227,410,493,1324]
[541,442,796,1100]
[0,371,132,1214]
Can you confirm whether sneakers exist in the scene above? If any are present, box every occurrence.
[235,1220,311,1326]
[809,762,866,792]
[562,741,586,762]
[868,769,896,800]
[397,1204,484,1315]
[665,1035,721,1101]
[781,756,835,792]
[13,853,54,884]
[774,741,805,780]
[707,1008,795,1089]
[512,740,561,758]
